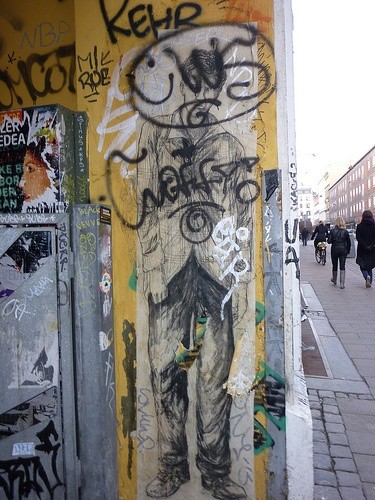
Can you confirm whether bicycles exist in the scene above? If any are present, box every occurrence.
[314,242,326,266]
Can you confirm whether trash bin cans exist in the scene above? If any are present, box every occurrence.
[346,228,357,259]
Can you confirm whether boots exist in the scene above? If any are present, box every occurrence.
[340,270,345,288]
[331,271,336,284]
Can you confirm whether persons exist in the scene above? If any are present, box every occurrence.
[327,216,352,289]
[311,220,329,263]
[356,210,375,288]
[301,226,309,246]
[19,137,60,203]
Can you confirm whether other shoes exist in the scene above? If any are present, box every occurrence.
[366,276,371,287]
